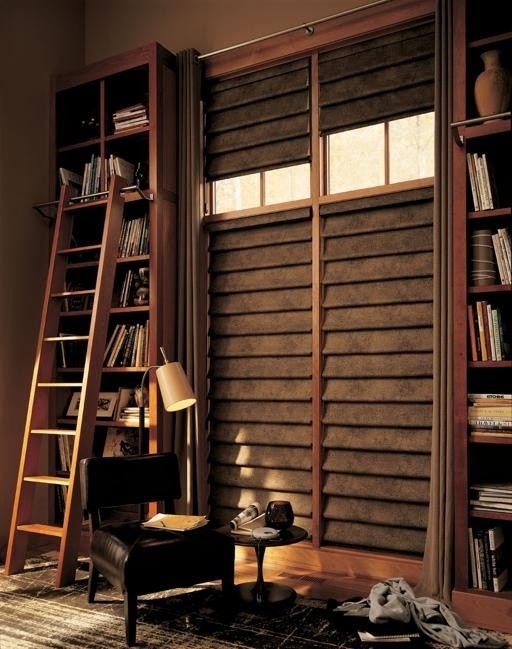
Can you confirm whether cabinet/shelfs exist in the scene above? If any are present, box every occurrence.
[450,0,511,635]
[48,42,179,556]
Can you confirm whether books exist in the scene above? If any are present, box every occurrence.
[465,152,511,594]
[356,622,421,649]
[229,502,268,535]
[142,513,211,531]
[58,103,149,527]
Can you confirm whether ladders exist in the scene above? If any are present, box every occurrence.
[4,174,127,587]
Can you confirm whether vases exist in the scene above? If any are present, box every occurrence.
[474,49,508,124]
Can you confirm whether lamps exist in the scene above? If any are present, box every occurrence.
[139,345,198,523]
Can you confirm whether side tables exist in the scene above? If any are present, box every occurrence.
[226,525,307,611]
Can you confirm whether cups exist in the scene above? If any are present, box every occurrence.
[266,500,294,529]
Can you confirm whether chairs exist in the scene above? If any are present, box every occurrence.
[80,454,235,647]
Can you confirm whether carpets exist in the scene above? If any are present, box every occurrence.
[0,547,511,649]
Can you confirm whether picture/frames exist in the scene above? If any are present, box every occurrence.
[113,386,136,421]
[62,390,120,421]
